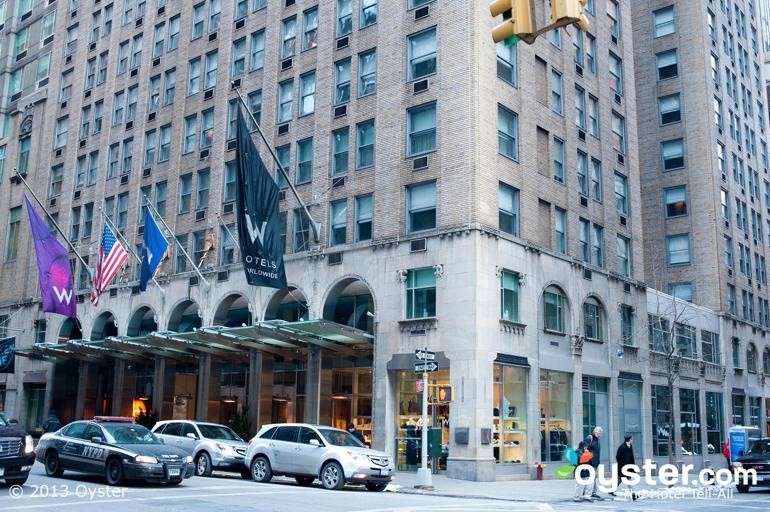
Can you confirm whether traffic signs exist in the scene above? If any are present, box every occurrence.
[414,361,440,374]
[414,349,436,361]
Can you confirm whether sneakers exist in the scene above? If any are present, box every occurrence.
[572,492,640,503]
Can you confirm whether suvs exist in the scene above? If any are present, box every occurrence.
[0,408,38,490]
[731,438,770,494]
[241,419,395,495]
[653,439,696,455]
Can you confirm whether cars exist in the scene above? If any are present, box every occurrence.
[143,417,253,480]
[541,444,567,460]
[33,413,196,490]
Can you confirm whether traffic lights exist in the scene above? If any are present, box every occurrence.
[436,384,455,404]
[549,0,589,33]
[489,0,536,45]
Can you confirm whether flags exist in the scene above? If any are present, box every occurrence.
[235,100,288,292]
[140,204,171,292]
[24,193,78,320]
[90,217,130,307]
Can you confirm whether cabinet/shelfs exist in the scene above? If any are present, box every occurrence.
[492,415,523,448]
[353,416,444,442]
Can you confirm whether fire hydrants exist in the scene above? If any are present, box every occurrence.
[706,466,716,485]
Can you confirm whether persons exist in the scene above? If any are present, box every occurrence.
[42,409,60,434]
[723,438,734,475]
[575,434,593,501]
[344,423,365,447]
[583,426,603,498]
[608,433,642,501]
[135,411,146,427]
